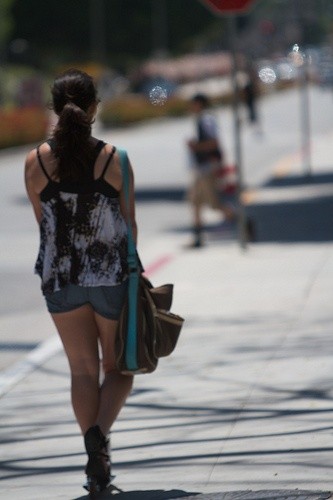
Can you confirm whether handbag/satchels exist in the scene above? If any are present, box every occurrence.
[116,273,185,375]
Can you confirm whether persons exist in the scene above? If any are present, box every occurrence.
[182,92,252,253]
[22,70,143,495]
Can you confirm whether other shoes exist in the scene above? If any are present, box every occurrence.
[82,478,111,499]
[84,425,108,491]
